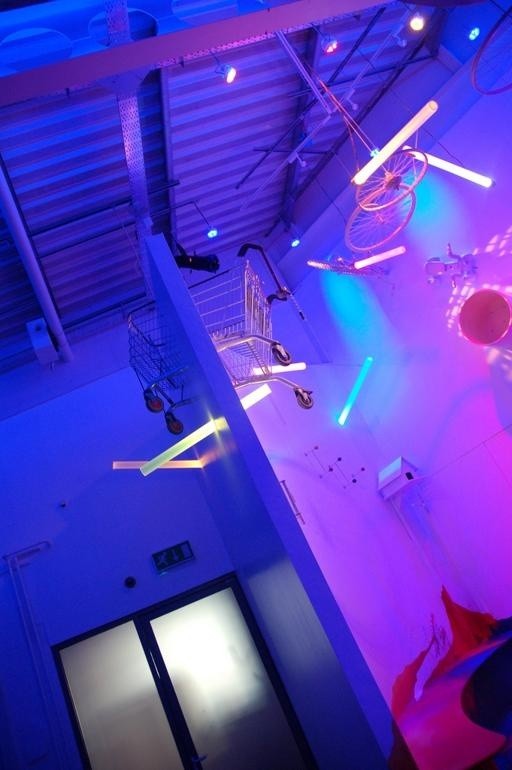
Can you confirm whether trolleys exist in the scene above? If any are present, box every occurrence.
[124,239,319,436]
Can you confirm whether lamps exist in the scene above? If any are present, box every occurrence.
[111,8,493,482]
